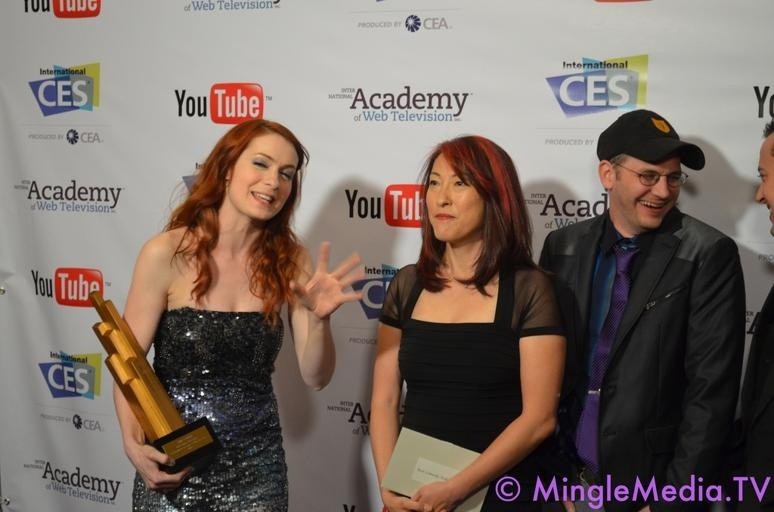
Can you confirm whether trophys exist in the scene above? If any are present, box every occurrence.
[90,291,219,471]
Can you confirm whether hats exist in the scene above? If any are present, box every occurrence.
[597,110,706,171]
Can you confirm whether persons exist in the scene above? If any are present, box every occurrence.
[368,134,568,512]
[537,111,747,510]
[113,119,365,512]
[723,121,774,512]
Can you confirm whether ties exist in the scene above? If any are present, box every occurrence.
[574,245,644,475]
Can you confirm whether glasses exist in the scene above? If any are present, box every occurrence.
[611,162,689,188]
